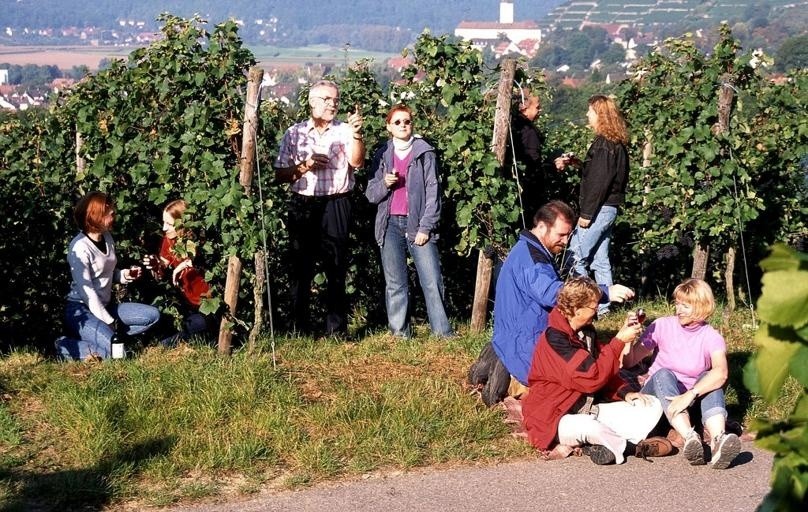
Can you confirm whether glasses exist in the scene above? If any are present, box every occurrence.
[387,118,412,127]
[586,306,599,312]
[315,97,340,106]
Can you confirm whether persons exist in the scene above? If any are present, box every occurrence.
[625,275,741,468]
[553,95,630,322]
[521,276,673,464]
[143,199,213,350]
[55,191,160,363]
[469,201,634,404]
[273,80,365,341]
[365,106,457,340]
[493,87,574,280]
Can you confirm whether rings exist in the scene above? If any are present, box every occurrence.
[315,160,317,164]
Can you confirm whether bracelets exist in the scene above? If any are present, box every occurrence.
[353,134,364,140]
[689,387,700,398]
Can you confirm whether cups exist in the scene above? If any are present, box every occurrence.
[127,266,139,277]
[148,254,158,267]
[627,307,646,325]
[390,167,399,177]
[562,151,575,165]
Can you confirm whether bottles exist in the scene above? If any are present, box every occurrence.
[108,317,127,361]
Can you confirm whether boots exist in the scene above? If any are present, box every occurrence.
[635,437,673,457]
[582,445,615,465]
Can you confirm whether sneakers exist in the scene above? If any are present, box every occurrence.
[710,432,741,469]
[467,341,514,407]
[683,432,705,467]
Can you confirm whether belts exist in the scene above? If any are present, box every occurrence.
[294,194,350,206]
